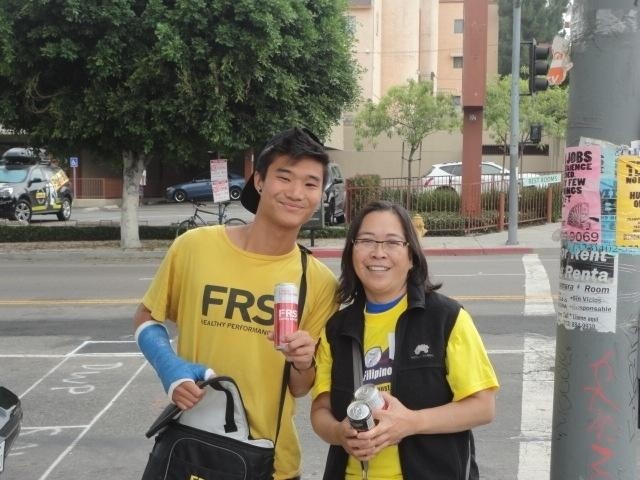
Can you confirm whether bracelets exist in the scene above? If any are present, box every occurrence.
[291,356,316,371]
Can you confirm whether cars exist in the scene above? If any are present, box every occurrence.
[300,162,347,230]
[418,162,549,199]
[164,170,246,203]
[0,148,73,223]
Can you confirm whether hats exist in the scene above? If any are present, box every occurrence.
[241,129,325,213]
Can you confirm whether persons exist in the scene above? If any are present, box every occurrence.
[131,127,343,480]
[308,199,501,480]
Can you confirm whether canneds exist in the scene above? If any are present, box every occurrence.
[354,384,388,425]
[348,401,378,432]
[274,283,299,351]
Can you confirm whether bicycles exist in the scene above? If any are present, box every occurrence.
[175,198,247,238]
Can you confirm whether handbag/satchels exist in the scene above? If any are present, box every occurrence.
[142,375,277,479]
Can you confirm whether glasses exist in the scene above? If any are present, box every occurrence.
[352,237,409,254]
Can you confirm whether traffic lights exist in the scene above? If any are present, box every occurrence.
[529,37,549,95]
